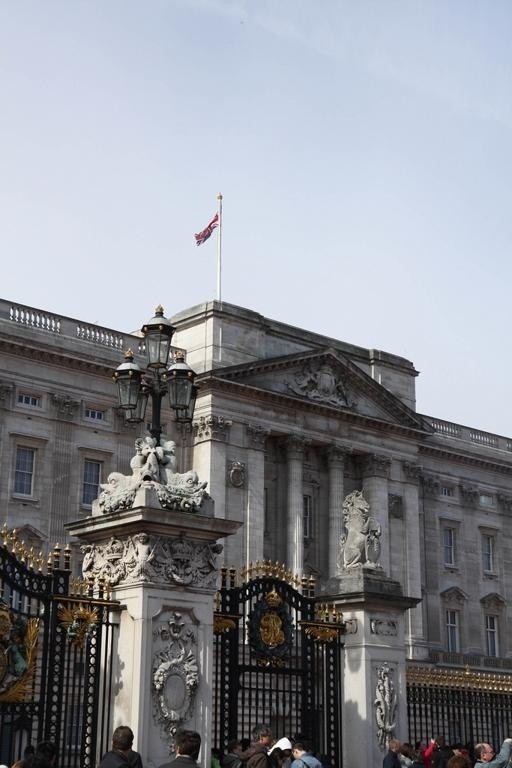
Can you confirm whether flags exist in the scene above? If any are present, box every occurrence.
[194,212,219,247]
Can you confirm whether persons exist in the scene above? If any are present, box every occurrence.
[97,726,143,768]
[212,724,331,768]
[0,740,56,768]
[159,730,201,768]
[383,736,512,768]
[129,532,159,579]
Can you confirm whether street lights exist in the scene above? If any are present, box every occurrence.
[112,307,199,448]
